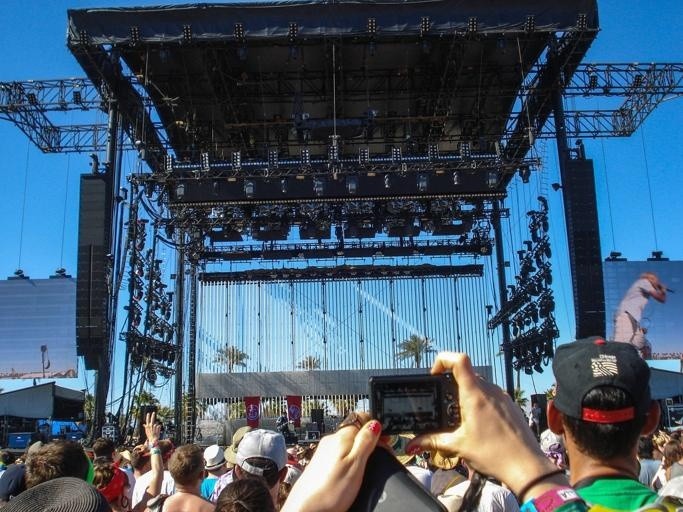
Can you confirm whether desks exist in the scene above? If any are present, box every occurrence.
[8,432,33,449]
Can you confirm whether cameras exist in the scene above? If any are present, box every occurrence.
[368,371,462,435]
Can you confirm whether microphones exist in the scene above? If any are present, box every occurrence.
[658,284,674,293]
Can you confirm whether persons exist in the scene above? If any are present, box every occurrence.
[0,336,683,512]
[613,273,666,342]
[632,328,648,350]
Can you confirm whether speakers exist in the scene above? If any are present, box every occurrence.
[311,409,324,433]
[530,394,548,432]
[141,405,157,441]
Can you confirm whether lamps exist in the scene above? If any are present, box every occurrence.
[312,175,326,197]
[175,179,186,200]
[337,242,345,256]
[487,170,498,191]
[242,178,258,200]
[415,171,430,193]
[488,182,561,374]
[451,170,464,186]
[279,176,288,194]
[344,174,359,194]
[382,173,392,190]
[133,178,177,383]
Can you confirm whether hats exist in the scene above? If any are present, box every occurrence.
[0,463,25,498]
[0,477,112,512]
[540,428,565,454]
[378,434,416,465]
[119,450,131,461]
[552,337,651,423]
[203,444,226,471]
[430,450,459,470]
[223,426,302,486]
